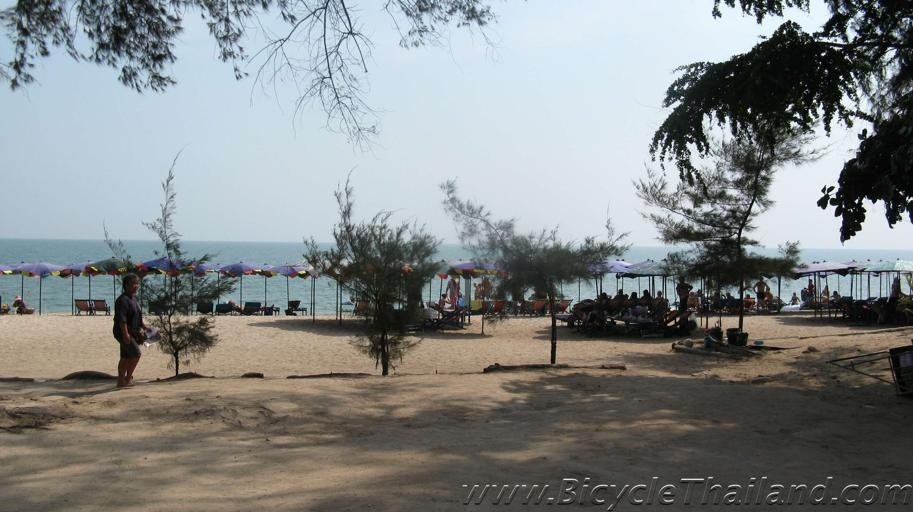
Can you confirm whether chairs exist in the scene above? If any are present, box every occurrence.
[73,298,111,316]
[195,297,261,315]
[426,297,572,330]
[575,294,693,336]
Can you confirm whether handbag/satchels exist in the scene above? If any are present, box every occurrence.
[132,328,161,345]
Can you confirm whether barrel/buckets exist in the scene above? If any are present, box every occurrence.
[737,333,748,345]
[889,343,912,394]
[727,328,741,344]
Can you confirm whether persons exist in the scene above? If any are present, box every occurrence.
[112,272,153,388]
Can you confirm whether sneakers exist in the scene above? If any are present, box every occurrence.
[117,374,134,385]
